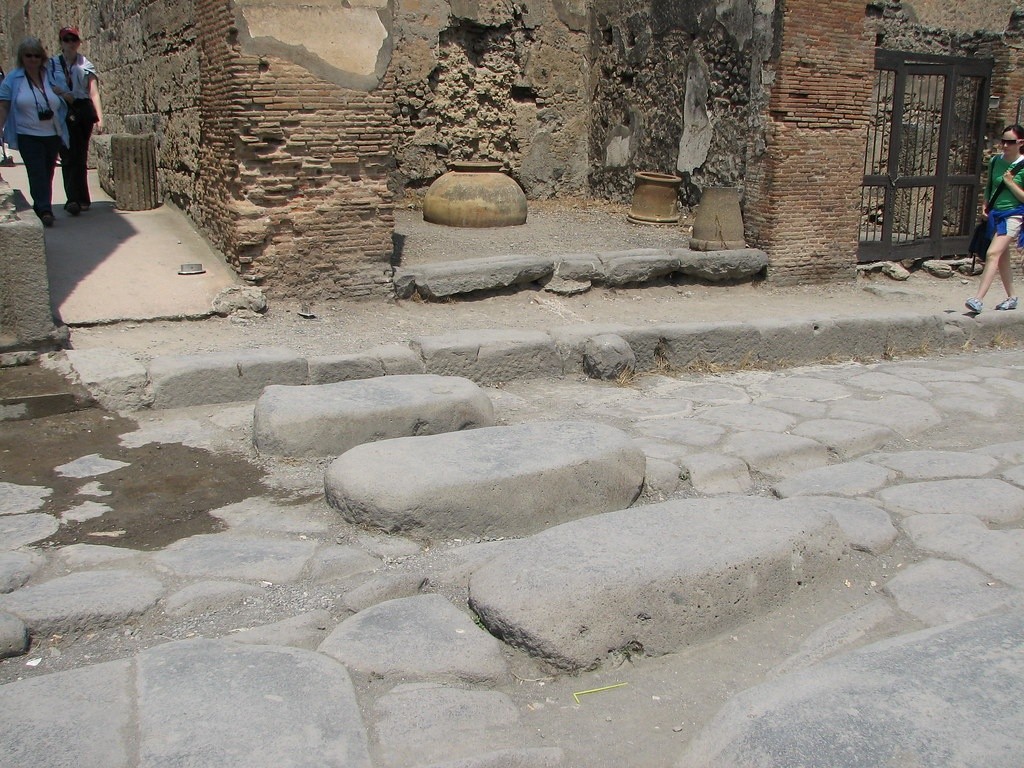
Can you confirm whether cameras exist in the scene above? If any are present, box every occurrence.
[38,109,54,121]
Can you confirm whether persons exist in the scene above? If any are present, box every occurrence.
[0,38,76,228]
[44,27,105,218]
[964,124,1024,314]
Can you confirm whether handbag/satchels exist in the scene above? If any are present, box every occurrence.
[74,98,99,124]
[968,218,987,260]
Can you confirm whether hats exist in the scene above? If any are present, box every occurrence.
[59,26,80,38]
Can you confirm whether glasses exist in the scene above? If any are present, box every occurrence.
[1000,138,1019,144]
[63,37,78,42]
[25,53,41,59]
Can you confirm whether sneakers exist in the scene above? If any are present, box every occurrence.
[964,299,983,312]
[77,201,91,210]
[996,296,1019,310]
[42,214,52,226]
[66,202,80,217]
